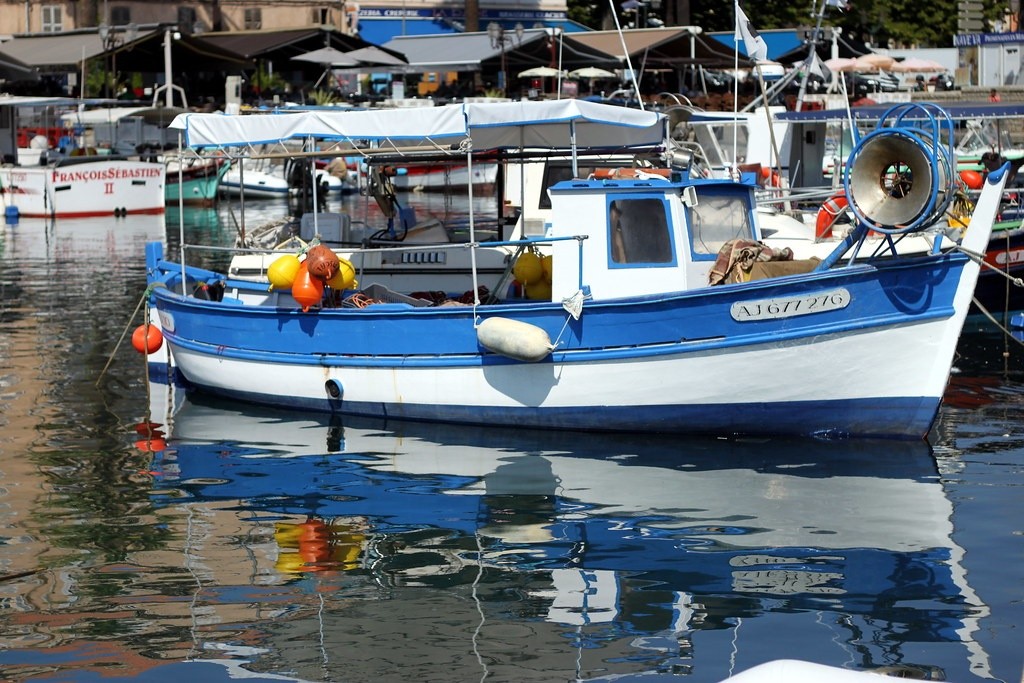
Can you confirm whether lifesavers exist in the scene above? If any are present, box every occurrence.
[815,188,878,240]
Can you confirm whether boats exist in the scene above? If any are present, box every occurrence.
[142,386,991,645]
[0,93,1024,440]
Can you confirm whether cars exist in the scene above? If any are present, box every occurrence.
[852,72,899,93]
